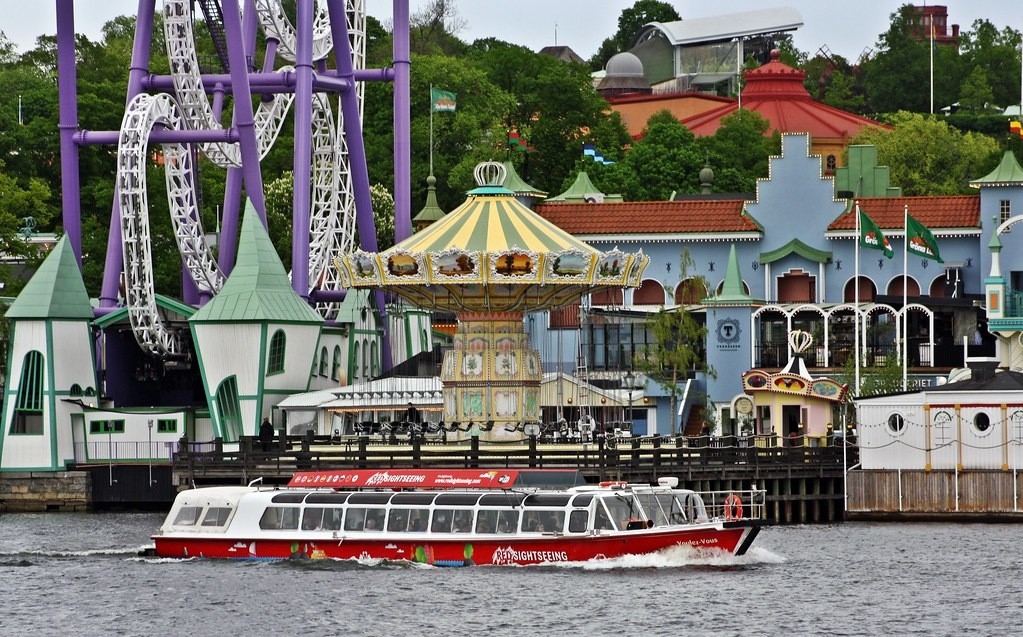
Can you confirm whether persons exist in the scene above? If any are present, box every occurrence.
[274,504,622,535]
[404,402,420,425]
[259,417,274,460]
[696,421,710,437]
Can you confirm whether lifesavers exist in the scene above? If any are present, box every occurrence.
[598,480,627,486]
[723,494,744,522]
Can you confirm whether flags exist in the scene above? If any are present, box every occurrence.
[432,87,456,113]
[858,209,894,259]
[907,213,944,263]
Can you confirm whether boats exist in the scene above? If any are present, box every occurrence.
[148,467,770,569]
